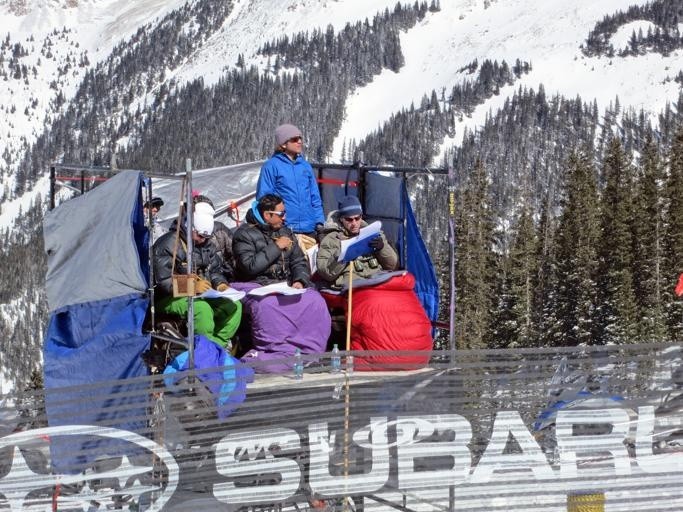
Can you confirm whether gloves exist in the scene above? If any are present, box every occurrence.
[216,283,228,291]
[196,280,210,294]
[368,237,384,250]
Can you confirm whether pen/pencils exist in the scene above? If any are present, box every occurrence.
[273,237,294,243]
[193,273,212,290]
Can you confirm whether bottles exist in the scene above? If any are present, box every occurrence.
[292,349,304,382]
[330,344,341,375]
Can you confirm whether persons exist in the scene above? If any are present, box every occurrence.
[142,186,169,247]
[314,194,399,291]
[170,194,238,282]
[151,202,243,348]
[230,193,311,290]
[255,124,325,252]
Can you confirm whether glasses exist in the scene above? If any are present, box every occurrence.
[265,210,286,217]
[288,136,302,143]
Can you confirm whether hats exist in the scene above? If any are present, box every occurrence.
[186,202,215,237]
[275,124,302,146]
[338,195,363,217]
